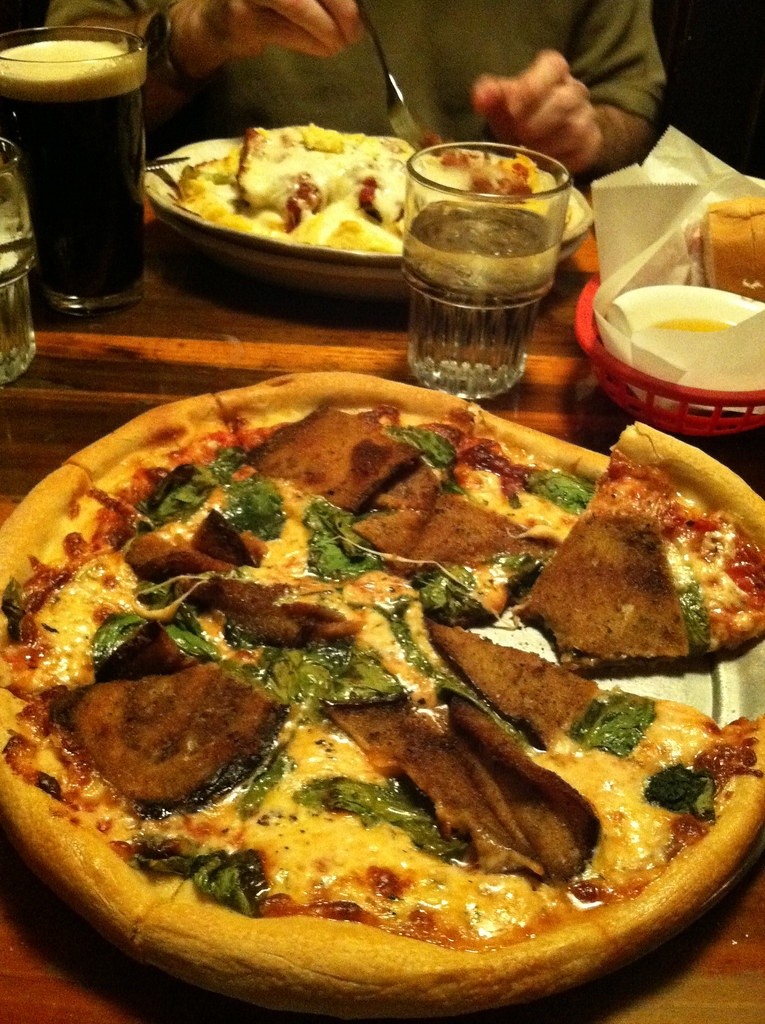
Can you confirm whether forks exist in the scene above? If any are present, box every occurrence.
[354,0,427,154]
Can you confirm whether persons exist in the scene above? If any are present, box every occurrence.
[47,1,669,182]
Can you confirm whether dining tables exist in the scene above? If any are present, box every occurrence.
[0,144,765,1024]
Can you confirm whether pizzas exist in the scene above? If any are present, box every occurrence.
[0,373,765,1016]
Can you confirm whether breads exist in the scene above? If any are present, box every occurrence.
[703,196,765,302]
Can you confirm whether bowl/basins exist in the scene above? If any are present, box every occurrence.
[608,284,765,330]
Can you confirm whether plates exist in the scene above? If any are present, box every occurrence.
[147,133,600,307]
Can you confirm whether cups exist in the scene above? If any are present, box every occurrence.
[0,25,146,317]
[0,138,40,386]
[400,141,575,403]
